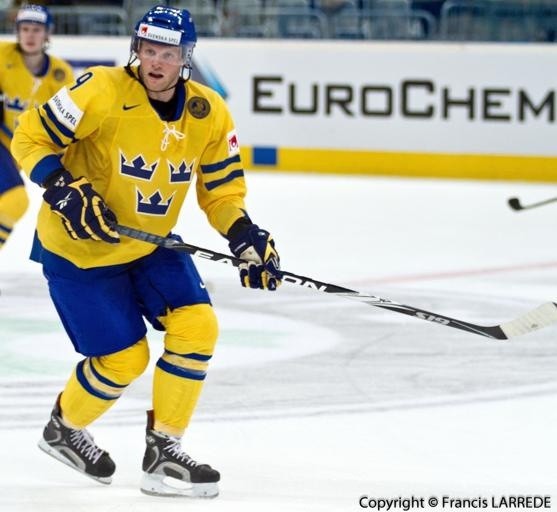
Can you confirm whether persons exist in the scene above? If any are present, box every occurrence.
[11,5,285,501]
[0,3,75,247]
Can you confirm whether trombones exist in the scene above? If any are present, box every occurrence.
[112,225,557,340]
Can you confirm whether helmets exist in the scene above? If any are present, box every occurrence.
[14,4,56,36]
[130,5,196,62]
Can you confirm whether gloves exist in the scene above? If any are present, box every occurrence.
[42,176,123,245]
[231,226,285,292]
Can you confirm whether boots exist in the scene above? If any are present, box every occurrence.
[43,391,116,478]
[142,411,221,484]
